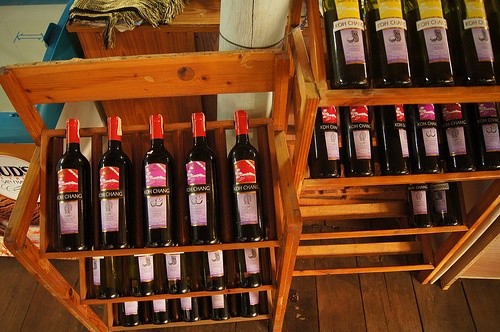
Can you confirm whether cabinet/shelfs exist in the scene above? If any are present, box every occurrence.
[0,0,500,332]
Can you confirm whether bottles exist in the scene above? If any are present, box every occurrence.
[449,0,500,86]
[437,103,477,172]
[98,117,132,249]
[322,0,372,90]
[88,247,264,299]
[118,291,260,327]
[466,103,500,170]
[429,183,462,226]
[406,104,443,175]
[405,183,434,227]
[184,111,222,245]
[310,106,342,178]
[340,106,375,177]
[228,110,268,242]
[142,115,173,248]
[364,0,413,87]
[374,104,411,175]
[402,0,456,88]
[56,119,91,251]
